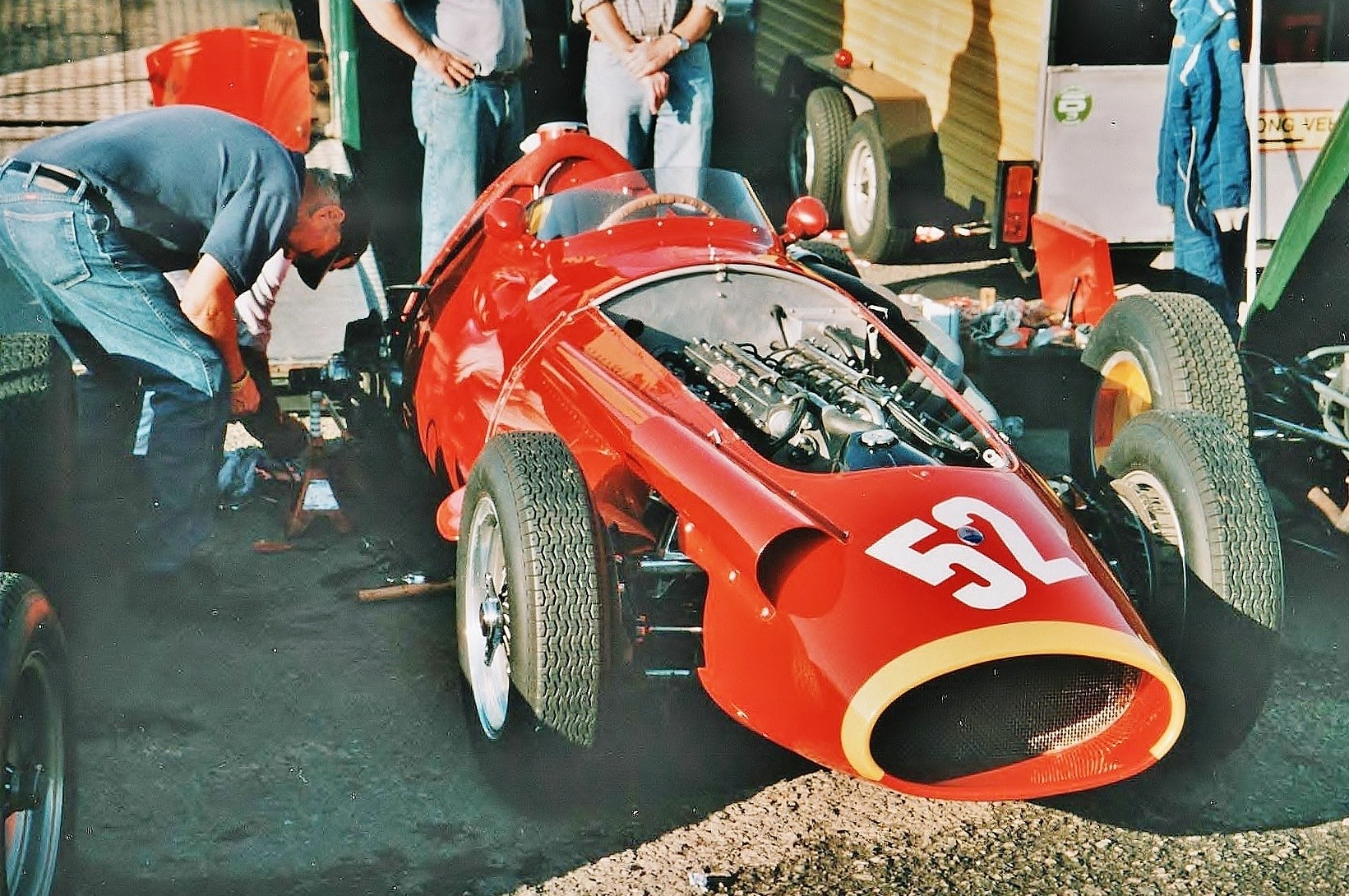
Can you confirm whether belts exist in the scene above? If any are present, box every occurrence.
[1,159,95,199]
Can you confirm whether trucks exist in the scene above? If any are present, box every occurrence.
[694,0,1348,271]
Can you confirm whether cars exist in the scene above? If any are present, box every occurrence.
[322,117,1285,819]
[1068,101,1349,496]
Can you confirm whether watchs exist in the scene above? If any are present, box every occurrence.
[667,32,690,50]
[232,370,249,393]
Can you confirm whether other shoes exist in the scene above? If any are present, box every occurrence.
[128,563,260,617]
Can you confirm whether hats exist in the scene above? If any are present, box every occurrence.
[297,174,372,290]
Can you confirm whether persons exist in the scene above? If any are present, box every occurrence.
[572,0,726,217]
[353,0,533,278]
[0,103,378,616]
